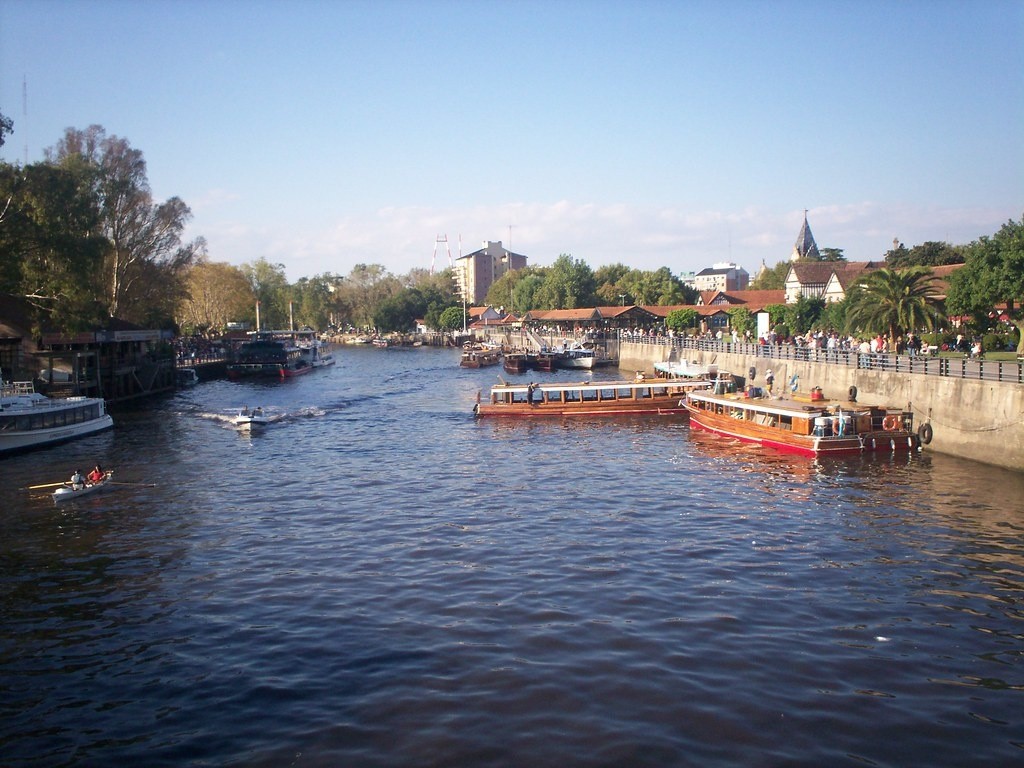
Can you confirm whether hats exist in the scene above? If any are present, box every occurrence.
[766,369,773,372]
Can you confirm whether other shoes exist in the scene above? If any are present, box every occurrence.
[769,398,773,401]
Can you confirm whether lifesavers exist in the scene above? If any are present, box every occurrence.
[749,367,756,379]
[848,386,857,397]
[831,417,848,435]
[918,423,933,444]
[882,415,897,431]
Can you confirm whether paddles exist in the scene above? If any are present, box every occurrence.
[84,479,157,489]
[18,480,74,492]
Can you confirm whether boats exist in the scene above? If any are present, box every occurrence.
[0,378,115,451]
[472,375,713,418]
[678,372,923,460]
[459,340,597,376]
[232,407,269,433]
[225,330,335,381]
[177,369,199,387]
[651,360,738,391]
[50,468,118,503]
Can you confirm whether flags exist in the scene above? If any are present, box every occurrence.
[789,373,799,391]
[838,409,844,437]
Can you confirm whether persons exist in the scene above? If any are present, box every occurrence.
[765,369,774,400]
[87,464,106,486]
[562,338,567,349]
[70,468,86,490]
[620,325,982,370]
